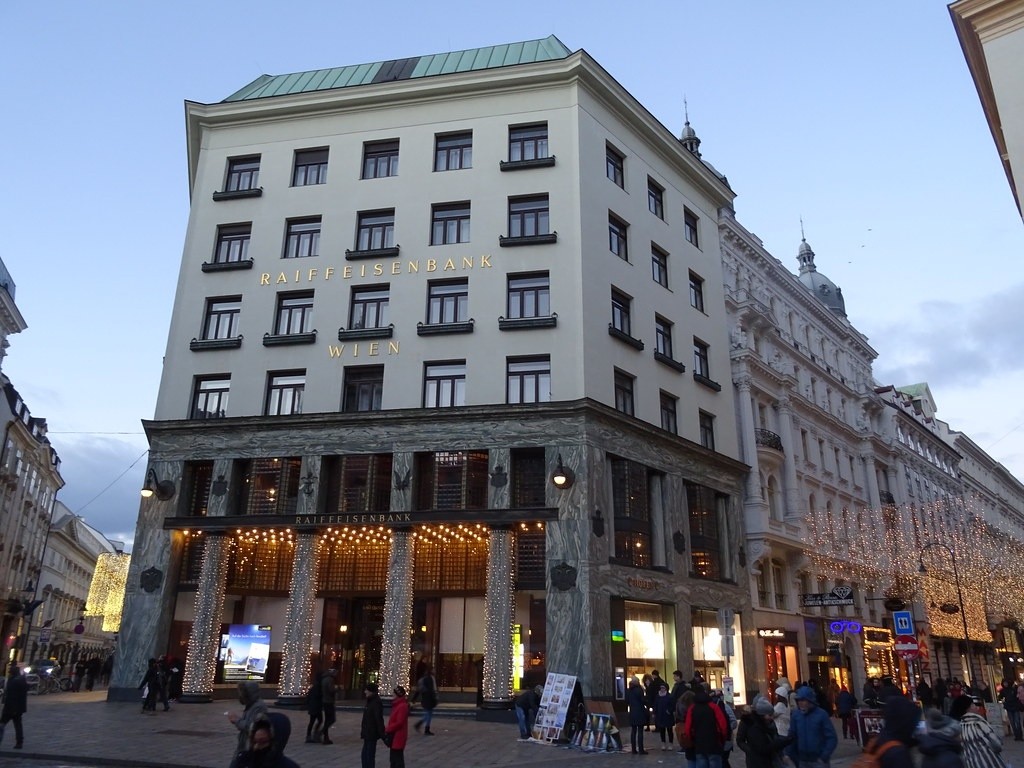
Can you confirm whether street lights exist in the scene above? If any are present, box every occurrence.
[918,543,977,688]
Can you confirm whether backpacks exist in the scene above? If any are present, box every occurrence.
[849,736,902,768]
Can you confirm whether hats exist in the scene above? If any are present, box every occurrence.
[949,695,973,719]
[752,693,763,707]
[367,683,379,693]
[756,697,773,715]
[394,686,407,697]
[775,682,789,701]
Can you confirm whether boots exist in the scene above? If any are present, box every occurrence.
[424,726,435,735]
[415,720,422,731]
[667,743,673,750]
[661,742,666,750]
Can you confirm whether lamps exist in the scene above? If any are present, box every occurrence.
[550,453,576,489]
[140,467,175,502]
[301,470,318,495]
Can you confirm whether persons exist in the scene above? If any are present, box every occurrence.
[71,646,114,692]
[229,712,300,768]
[808,678,1008,768]
[411,667,438,736]
[360,683,385,768]
[137,655,185,714]
[515,685,544,739]
[385,685,410,768]
[626,677,648,755]
[0,666,27,749]
[227,681,268,760]
[736,696,793,768]
[411,655,432,703]
[304,671,323,743]
[997,678,1024,741]
[770,686,790,743]
[775,677,792,693]
[314,666,340,744]
[642,670,739,768]
[784,686,837,768]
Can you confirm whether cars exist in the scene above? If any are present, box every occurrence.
[24,659,61,676]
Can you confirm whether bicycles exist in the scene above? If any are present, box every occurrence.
[23,669,73,695]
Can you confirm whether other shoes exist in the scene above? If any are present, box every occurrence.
[518,735,540,742]
[842,732,848,739]
[645,727,650,731]
[1014,734,1024,741]
[850,734,854,739]
[13,744,23,749]
[676,749,685,754]
[652,728,659,732]
[639,747,649,754]
[632,747,638,753]
[305,730,334,745]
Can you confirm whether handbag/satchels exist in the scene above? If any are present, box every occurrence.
[421,696,436,710]
[141,686,149,699]
[382,730,396,747]
[675,722,693,749]
